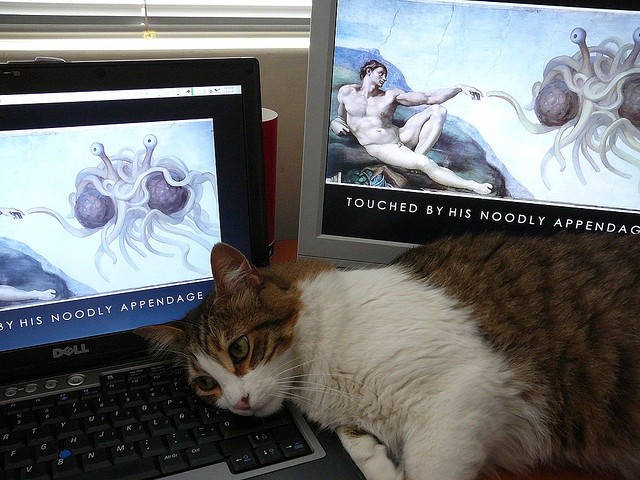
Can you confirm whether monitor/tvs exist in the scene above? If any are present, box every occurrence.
[297,0,640,272]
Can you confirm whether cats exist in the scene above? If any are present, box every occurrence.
[128,222,640,480]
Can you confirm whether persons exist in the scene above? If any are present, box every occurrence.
[330,59,493,196]
[0,206,57,301]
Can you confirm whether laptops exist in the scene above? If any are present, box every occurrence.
[1,56,366,480]
